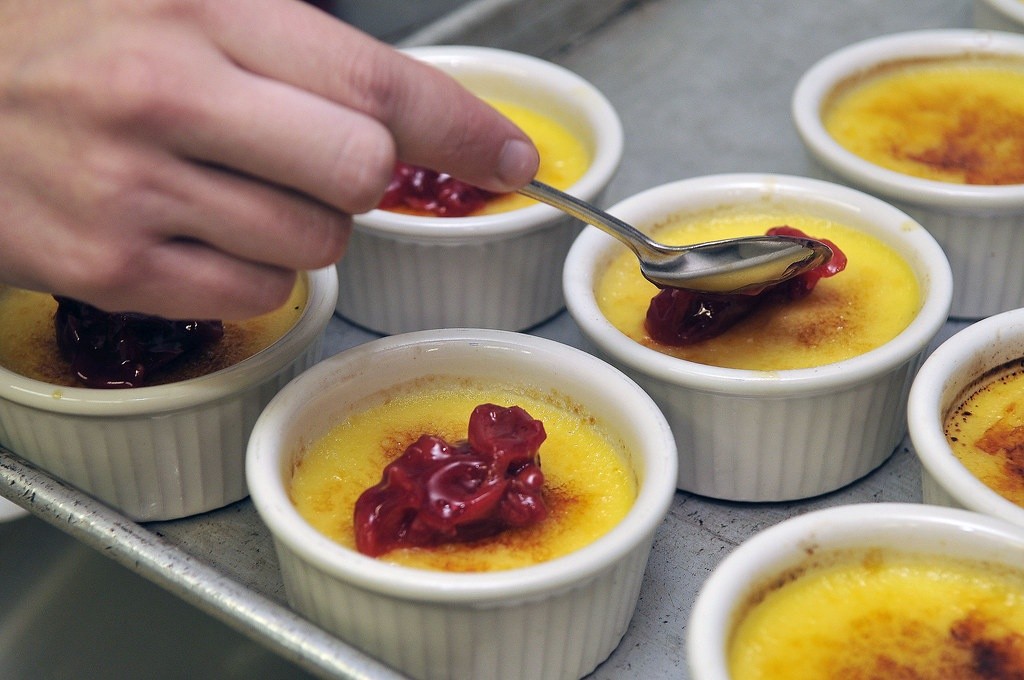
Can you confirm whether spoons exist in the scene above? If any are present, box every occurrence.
[516,179,833,290]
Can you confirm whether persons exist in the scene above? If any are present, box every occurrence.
[0,0,541,323]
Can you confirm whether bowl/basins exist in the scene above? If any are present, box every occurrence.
[0,264,342,523]
[793,29,1024,320]
[905,305,1024,533]
[325,45,626,337]
[245,327,677,680]
[684,503,1024,680]
[565,174,952,503]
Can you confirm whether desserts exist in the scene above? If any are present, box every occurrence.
[0,53,1024,680]
